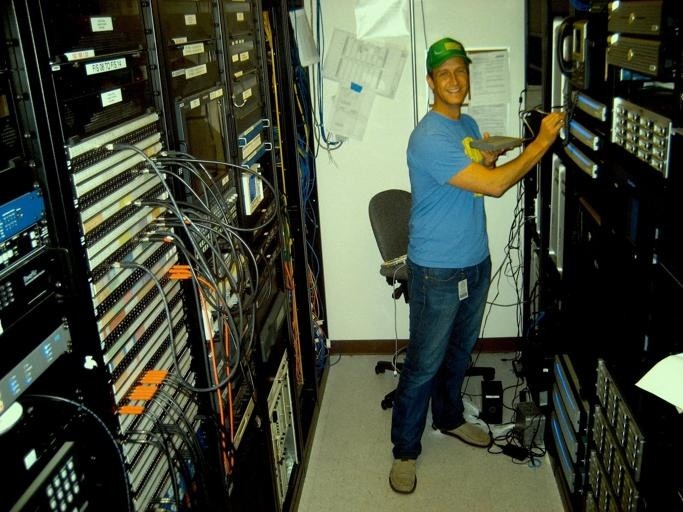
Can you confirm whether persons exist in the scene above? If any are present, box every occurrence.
[390,37,565,494]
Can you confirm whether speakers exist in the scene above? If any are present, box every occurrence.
[482,381,502,424]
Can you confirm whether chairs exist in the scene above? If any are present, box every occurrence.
[368,189,494,410]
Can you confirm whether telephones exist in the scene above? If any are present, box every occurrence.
[557,16,604,91]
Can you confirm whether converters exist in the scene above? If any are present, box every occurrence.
[516,403,546,446]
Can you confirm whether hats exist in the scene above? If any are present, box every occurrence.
[426,38,472,73]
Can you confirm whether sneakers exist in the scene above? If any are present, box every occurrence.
[441,423,491,448]
[389,459,417,494]
